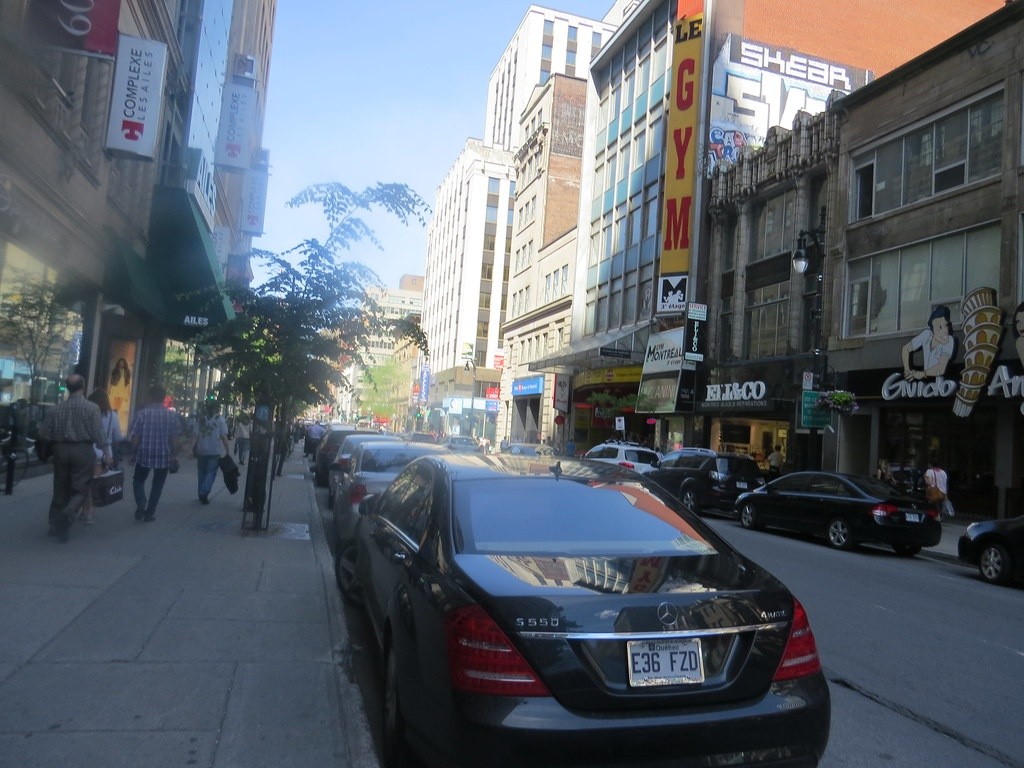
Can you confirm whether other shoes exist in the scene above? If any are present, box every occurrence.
[144,514,156,521]
[134,509,146,520]
[198,494,210,504]
[48,522,72,542]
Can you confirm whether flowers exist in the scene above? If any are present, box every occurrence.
[815,390,859,416]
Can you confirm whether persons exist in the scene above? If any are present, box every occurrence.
[38,373,114,544]
[874,455,893,489]
[500,436,507,451]
[124,385,184,522]
[194,401,231,507]
[108,358,130,410]
[566,439,576,457]
[305,420,326,459]
[922,456,948,523]
[82,387,121,523]
[473,434,492,455]
[767,446,783,475]
[237,410,301,464]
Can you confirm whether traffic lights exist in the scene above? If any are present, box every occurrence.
[416,413,421,417]
[355,416,360,420]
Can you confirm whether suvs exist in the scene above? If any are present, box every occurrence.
[641,448,769,517]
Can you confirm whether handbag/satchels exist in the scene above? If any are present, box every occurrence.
[93,462,124,507]
[926,485,944,505]
[941,495,954,519]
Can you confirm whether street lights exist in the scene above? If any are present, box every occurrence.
[392,381,399,432]
[462,355,476,436]
[793,204,827,471]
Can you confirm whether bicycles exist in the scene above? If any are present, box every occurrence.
[0,439,30,491]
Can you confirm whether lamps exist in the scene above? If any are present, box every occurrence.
[101,303,125,317]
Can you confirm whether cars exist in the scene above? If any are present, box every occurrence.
[585,439,668,474]
[957,512,1024,585]
[338,454,833,768]
[309,423,491,543]
[734,470,942,556]
[502,443,575,461]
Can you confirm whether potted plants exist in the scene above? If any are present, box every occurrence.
[585,392,618,418]
[618,393,638,412]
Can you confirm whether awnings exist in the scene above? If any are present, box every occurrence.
[112,185,239,343]
